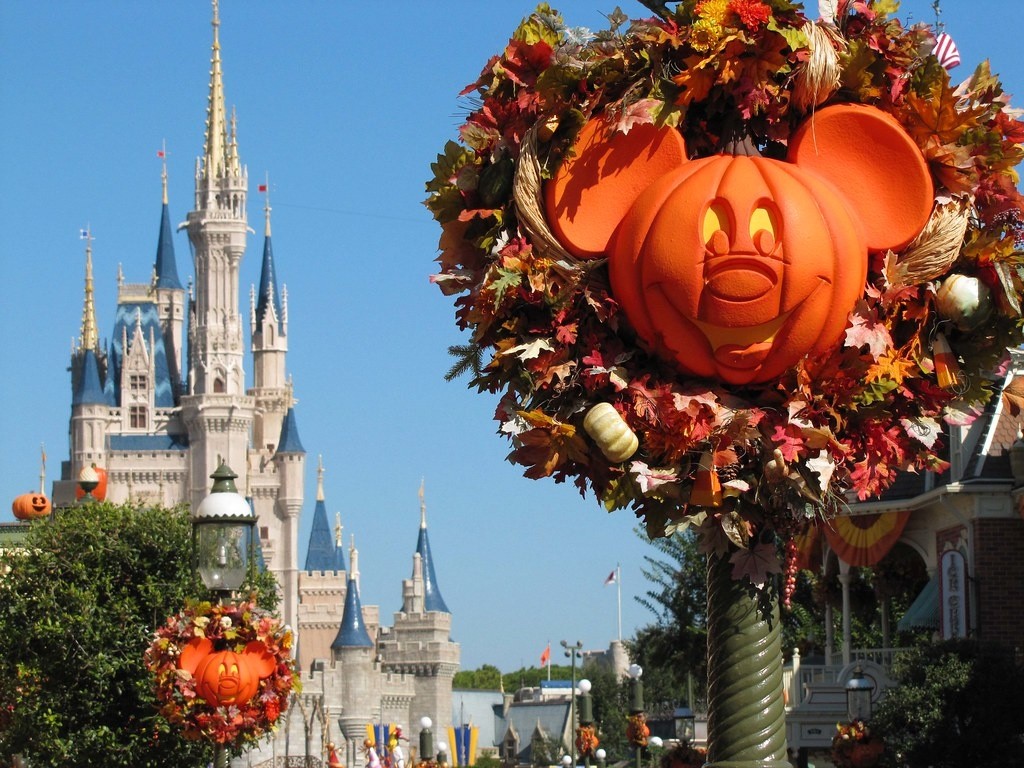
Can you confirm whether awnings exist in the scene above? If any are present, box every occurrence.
[898,572,939,630]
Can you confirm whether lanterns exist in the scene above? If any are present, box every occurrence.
[12,492,51,520]
[844,739,885,768]
[545,103,934,385]
[178,638,276,709]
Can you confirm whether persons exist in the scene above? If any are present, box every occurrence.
[388,725,410,768]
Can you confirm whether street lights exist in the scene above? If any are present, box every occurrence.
[420,716,435,762]
[578,678,594,768]
[594,748,607,768]
[438,741,448,763]
[190,458,260,768]
[628,663,646,768]
[559,639,583,768]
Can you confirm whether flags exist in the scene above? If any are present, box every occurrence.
[158,151,163,156]
[931,32,961,70]
[81,230,87,239]
[541,647,549,666]
[259,185,266,191]
[606,570,616,584]
